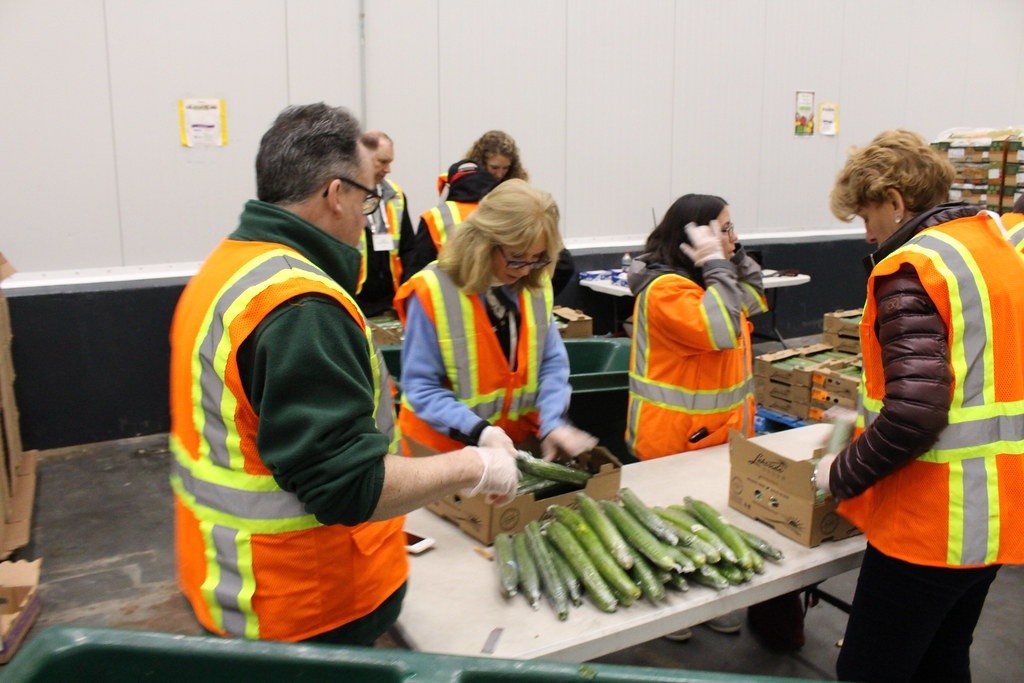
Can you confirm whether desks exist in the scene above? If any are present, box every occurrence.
[578,267,811,349]
[392,422,867,663]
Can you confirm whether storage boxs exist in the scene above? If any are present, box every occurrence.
[365,313,404,347]
[552,306,595,339]
[929,127,1024,216]
[422,444,623,547]
[753,306,864,424]
[0,257,43,665]
[728,426,868,548]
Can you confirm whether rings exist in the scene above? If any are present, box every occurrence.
[810,468,818,491]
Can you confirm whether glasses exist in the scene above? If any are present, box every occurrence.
[718,223,735,233]
[497,245,551,269]
[323,178,383,215]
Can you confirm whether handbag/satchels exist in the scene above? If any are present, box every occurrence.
[748,592,806,650]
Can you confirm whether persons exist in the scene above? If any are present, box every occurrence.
[388,177,600,464]
[344,129,416,321]
[623,193,768,464]
[438,129,531,202]
[807,125,1024,683]
[166,101,524,654]
[409,158,504,288]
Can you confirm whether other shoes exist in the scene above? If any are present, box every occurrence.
[706,609,741,633]
[666,626,693,643]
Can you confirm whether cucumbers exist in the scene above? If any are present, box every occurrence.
[515,449,594,497]
[500,488,784,621]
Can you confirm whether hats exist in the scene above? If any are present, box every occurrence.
[446,157,487,187]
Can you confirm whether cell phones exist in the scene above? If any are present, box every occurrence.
[402,531,435,553]
[690,427,709,443]
